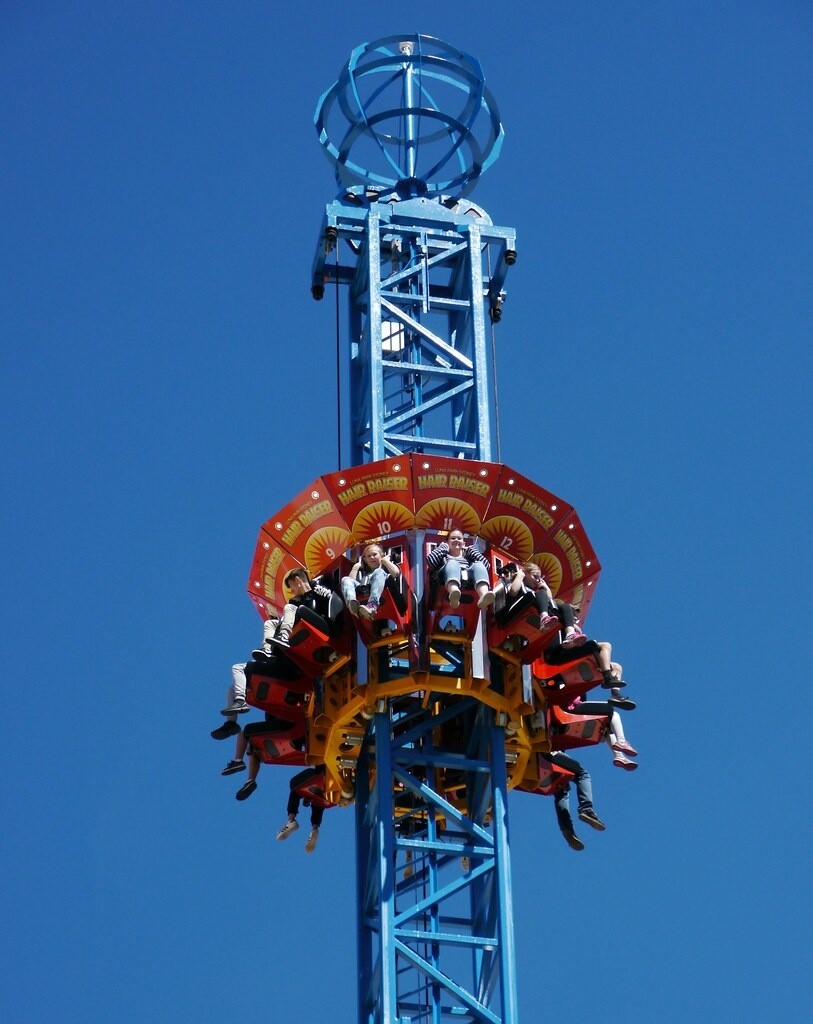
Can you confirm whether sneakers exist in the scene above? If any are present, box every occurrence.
[305,829,317,852]
[347,599,360,619]
[265,633,290,650]
[359,603,377,621]
[612,739,638,757]
[277,820,299,841]
[562,634,587,650]
[252,648,277,663]
[539,615,558,633]
[614,752,639,771]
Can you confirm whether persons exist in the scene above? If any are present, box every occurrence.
[212,564,637,880]
[426,529,495,608]
[341,544,400,622]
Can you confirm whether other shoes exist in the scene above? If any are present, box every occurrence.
[211,721,241,740]
[608,694,636,710]
[220,702,250,716]
[461,856,469,871]
[601,673,627,689]
[405,857,414,880]
[579,809,607,831]
[449,589,461,608]
[563,829,584,851]
[221,760,246,776]
[236,780,257,801]
[477,591,495,608]
